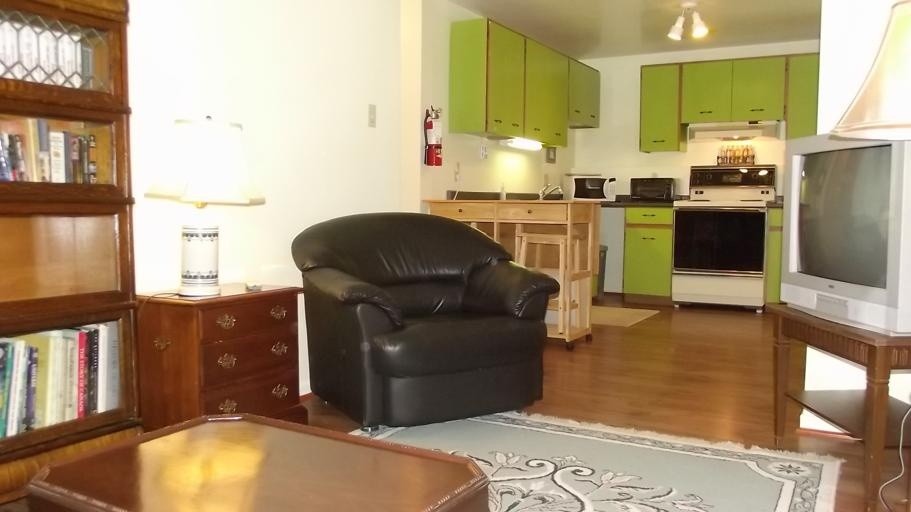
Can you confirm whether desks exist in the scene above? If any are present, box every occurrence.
[26,410,490,512]
[423,199,602,351]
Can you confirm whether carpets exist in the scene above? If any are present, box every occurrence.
[349,408,847,512]
[591,305,661,329]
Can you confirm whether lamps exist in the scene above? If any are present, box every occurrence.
[829,0,911,142]
[144,115,265,296]
[667,8,685,41]
[500,137,542,152]
[691,6,709,39]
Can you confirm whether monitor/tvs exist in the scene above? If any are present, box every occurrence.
[779,133,910,336]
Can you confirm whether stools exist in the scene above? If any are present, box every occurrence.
[516,232,582,334]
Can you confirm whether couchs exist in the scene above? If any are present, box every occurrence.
[290,212,561,433]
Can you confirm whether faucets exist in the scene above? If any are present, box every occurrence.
[538,183,564,201]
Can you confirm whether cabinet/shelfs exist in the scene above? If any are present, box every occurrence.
[0,0,145,506]
[765,301,911,512]
[135,280,309,433]
[732,54,787,123]
[638,62,687,153]
[764,203,783,308]
[448,18,526,140]
[680,59,733,124]
[524,37,569,148]
[623,202,673,305]
[785,53,820,141]
[569,56,601,129]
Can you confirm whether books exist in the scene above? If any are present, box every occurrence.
[0,321,119,441]
[0,118,116,184]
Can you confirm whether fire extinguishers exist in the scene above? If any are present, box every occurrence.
[424,105,443,166]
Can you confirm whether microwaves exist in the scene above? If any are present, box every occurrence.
[571,176,616,201]
[630,178,674,201]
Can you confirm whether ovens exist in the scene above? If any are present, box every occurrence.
[671,200,767,306]
